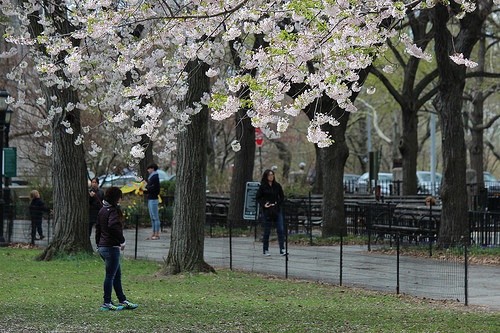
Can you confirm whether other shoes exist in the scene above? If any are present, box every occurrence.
[280,250,289,255]
[151,236,160,239]
[263,250,269,256]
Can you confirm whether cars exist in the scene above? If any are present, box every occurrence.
[483,171,500,194]
[342,174,361,195]
[98,176,136,188]
[416,171,443,198]
[354,171,395,198]
[308,168,317,186]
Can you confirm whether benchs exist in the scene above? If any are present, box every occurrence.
[372,203,442,250]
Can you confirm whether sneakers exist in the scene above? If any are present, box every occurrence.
[100,303,118,311]
[118,301,138,310]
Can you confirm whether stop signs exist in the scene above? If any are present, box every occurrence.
[255,129,265,147]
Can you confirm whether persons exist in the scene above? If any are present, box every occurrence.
[87,176,105,253]
[296,162,308,175]
[254,168,290,258]
[425,195,437,207]
[270,166,279,174]
[143,162,163,240]
[374,185,382,203]
[27,189,45,243]
[94,186,139,312]
